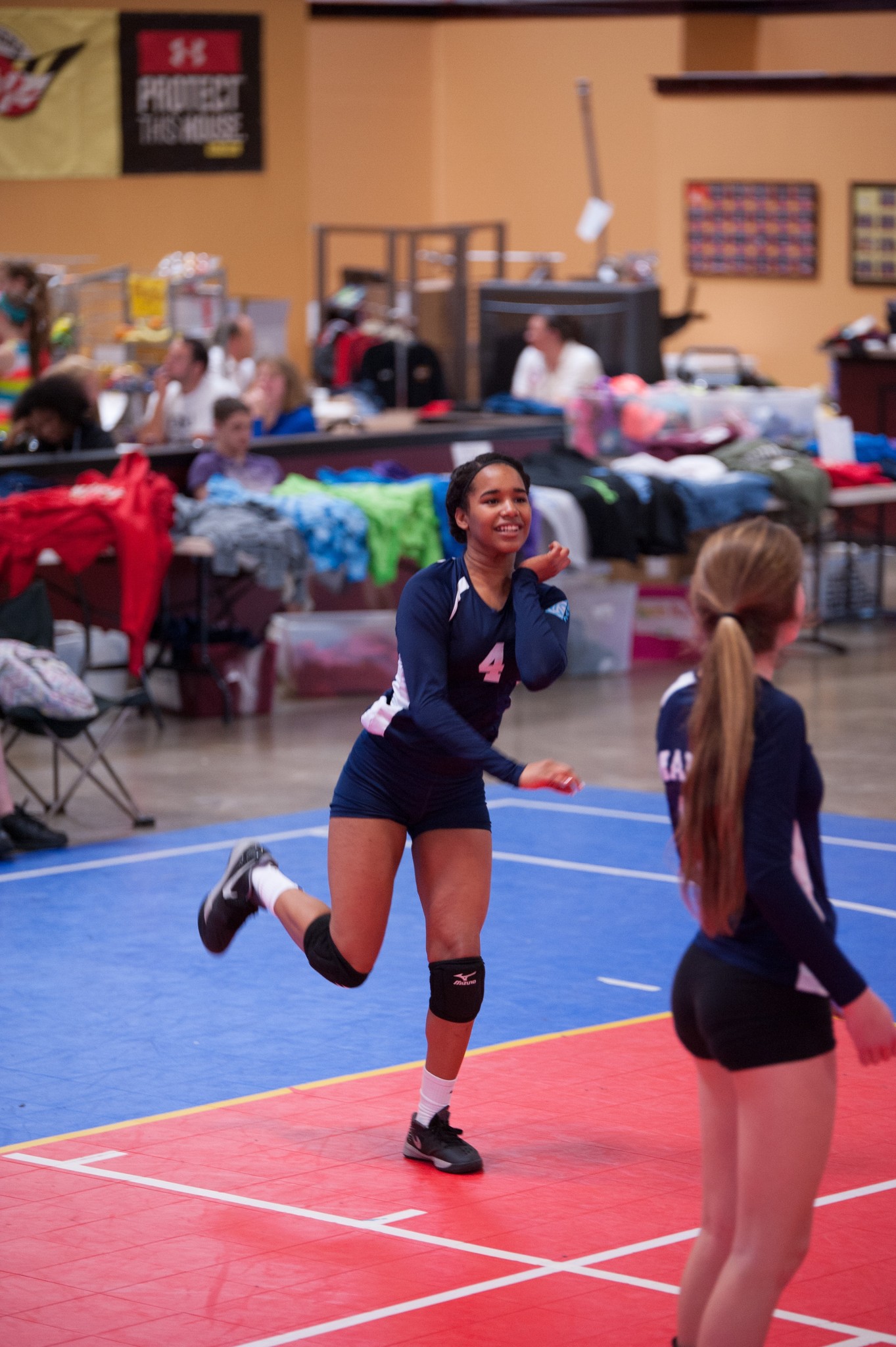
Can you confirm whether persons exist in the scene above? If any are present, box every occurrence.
[0,264,319,498]
[656,515,896,1347]
[511,315,603,405]
[196,451,584,1175]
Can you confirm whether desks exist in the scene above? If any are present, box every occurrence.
[796,484,896,654]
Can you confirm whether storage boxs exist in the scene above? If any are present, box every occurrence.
[545,577,638,676]
[273,609,398,699]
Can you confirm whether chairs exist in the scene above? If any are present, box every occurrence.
[0,579,157,835]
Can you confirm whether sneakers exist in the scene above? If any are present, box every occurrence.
[197,837,279,953]
[403,1104,485,1174]
[0,798,69,862]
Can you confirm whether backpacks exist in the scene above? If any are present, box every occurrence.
[1,635,102,719]
